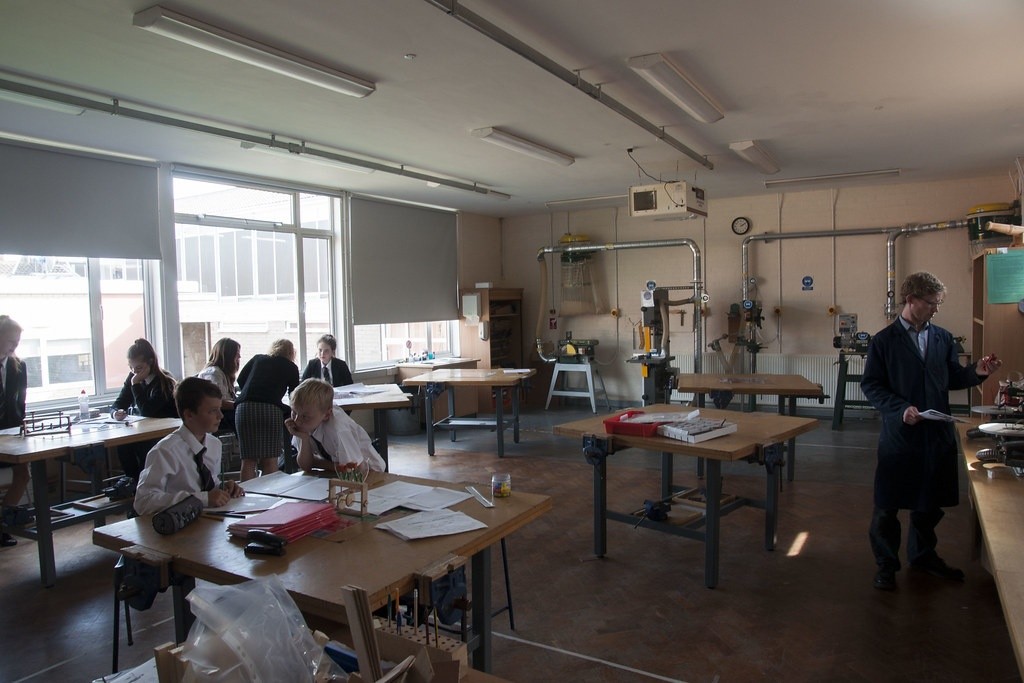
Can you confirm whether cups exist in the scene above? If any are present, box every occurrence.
[492,473,511,497]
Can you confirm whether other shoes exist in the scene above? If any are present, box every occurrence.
[105,477,136,497]
[0,531,17,546]
[127,505,139,519]
[1,506,34,528]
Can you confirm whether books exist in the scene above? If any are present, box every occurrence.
[82,412,147,424]
[203,469,478,545]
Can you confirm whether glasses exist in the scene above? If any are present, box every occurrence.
[912,294,945,306]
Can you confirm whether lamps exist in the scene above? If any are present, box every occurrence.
[628,53,726,125]
[472,128,575,169]
[133,7,377,102]
[240,140,376,177]
[0,89,88,118]
[764,169,902,189]
[730,140,781,177]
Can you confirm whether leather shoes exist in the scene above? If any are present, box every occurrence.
[876,571,897,591]
[916,558,965,582]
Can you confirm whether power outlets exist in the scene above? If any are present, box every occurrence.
[626,147,634,156]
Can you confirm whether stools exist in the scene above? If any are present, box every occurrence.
[422,536,515,644]
[110,551,134,675]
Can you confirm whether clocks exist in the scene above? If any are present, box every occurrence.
[733,218,750,236]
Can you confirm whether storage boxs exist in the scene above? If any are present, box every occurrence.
[603,411,674,438]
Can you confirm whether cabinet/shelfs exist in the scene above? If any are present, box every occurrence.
[397,363,479,426]
[971,246,1024,419]
[460,288,525,413]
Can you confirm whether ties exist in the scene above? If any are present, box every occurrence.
[323,367,331,384]
[311,435,332,463]
[194,447,215,492]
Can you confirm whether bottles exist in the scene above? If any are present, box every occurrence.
[78,389,91,421]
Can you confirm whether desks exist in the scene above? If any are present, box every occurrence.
[403,369,538,459]
[954,418,1024,683]
[545,362,612,415]
[236,384,413,473]
[0,413,187,588]
[678,373,824,483]
[93,468,552,682]
[551,403,822,589]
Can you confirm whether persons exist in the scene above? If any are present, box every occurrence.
[283,377,392,477]
[191,336,242,441]
[133,374,247,516]
[299,333,357,416]
[109,336,178,498]
[0,315,30,548]
[861,270,1006,593]
[234,338,300,486]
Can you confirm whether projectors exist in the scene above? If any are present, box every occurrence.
[628,181,707,222]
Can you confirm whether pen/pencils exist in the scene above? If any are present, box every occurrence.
[108,406,130,418]
[223,514,245,519]
[199,513,224,521]
[222,463,225,490]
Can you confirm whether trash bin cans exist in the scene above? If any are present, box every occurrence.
[383,381,422,436]
